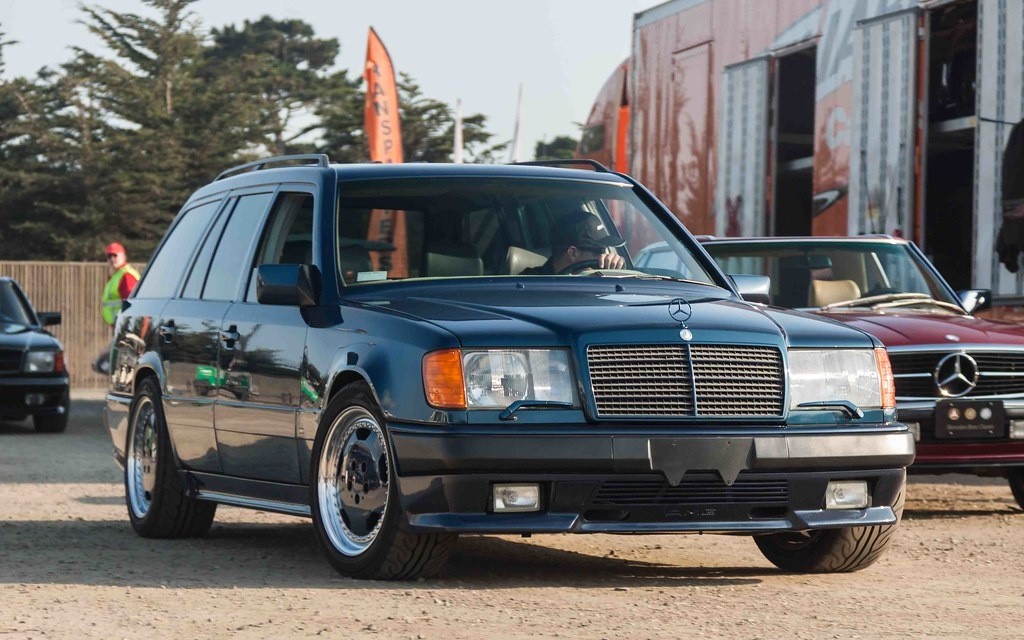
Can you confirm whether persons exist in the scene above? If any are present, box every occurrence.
[517,210,627,276]
[101,243,143,338]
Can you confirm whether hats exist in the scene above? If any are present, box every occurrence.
[550,211,627,249]
[105,242,125,257]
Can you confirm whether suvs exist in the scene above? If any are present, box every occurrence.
[633,232,1024,515]
[102,153,918,583]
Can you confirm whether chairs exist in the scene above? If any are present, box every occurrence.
[808,279,862,307]
[303,245,372,284]
[425,252,485,277]
[499,246,549,275]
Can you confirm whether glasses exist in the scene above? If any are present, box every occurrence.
[576,248,607,255]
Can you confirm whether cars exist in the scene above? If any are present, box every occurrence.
[0,274,71,434]
